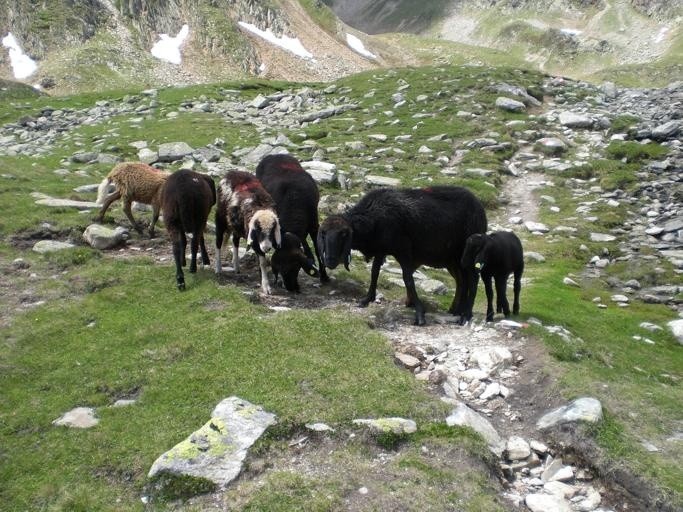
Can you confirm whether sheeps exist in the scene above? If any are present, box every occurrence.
[315,183,489,325]
[255,153,332,294]
[455,231,525,321]
[160,168,216,293]
[212,169,283,294]
[98,159,166,240]
